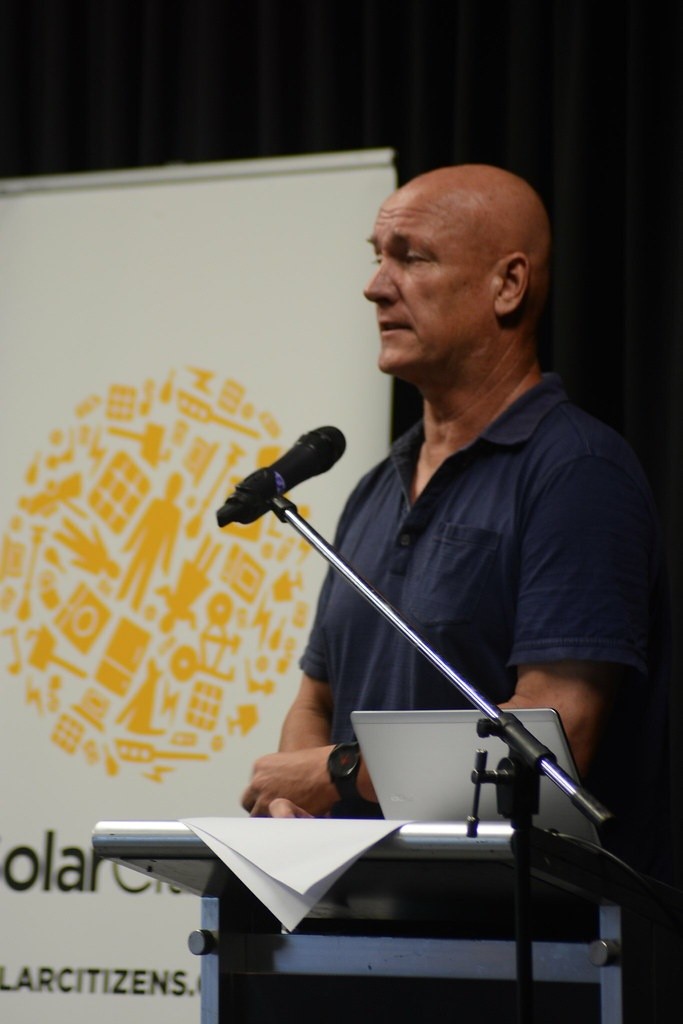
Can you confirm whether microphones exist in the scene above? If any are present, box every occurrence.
[216,427,346,528]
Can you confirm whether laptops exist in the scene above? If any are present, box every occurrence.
[350,707,604,858]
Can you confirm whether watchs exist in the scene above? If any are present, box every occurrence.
[327,742,361,801]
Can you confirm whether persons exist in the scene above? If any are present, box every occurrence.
[241,163,676,889]
